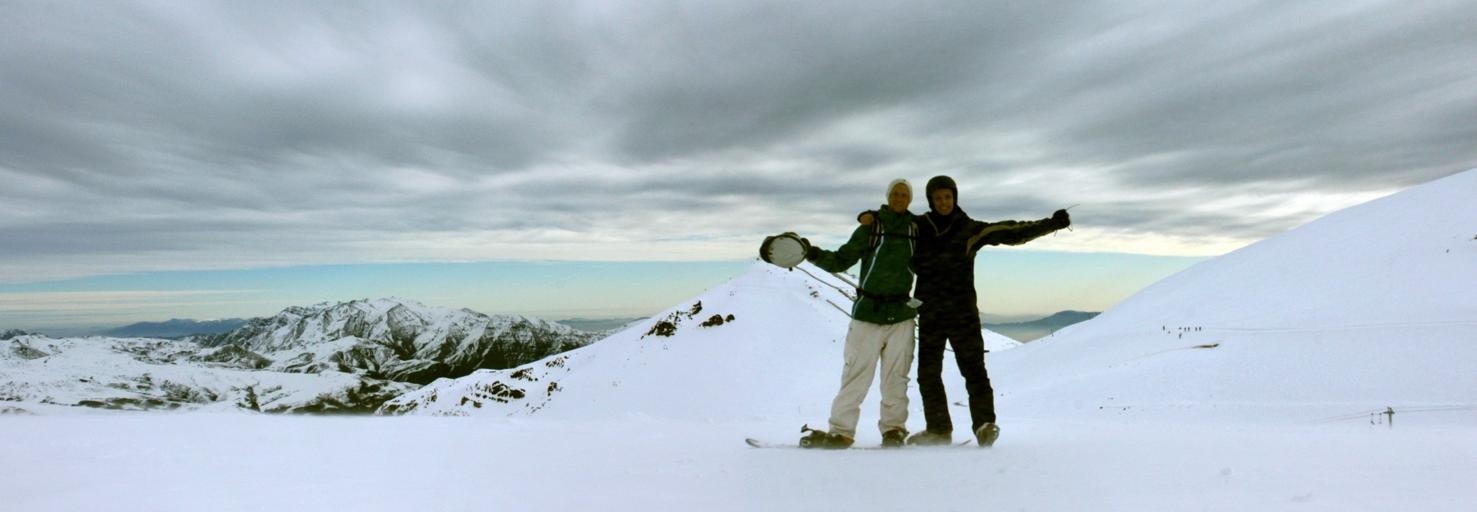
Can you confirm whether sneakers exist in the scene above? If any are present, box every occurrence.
[904,427,952,447]
[800,429,853,448]
[976,422,999,447]
[880,426,909,446]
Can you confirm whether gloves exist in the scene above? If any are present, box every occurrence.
[1052,209,1072,230]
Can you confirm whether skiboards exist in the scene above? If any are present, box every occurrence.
[746,438,970,449]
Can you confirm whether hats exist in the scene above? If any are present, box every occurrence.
[925,175,959,211]
[886,179,912,204]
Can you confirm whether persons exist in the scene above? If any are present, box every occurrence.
[857,175,1070,448]
[799,179,920,447]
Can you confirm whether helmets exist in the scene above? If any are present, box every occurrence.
[759,232,809,272]
